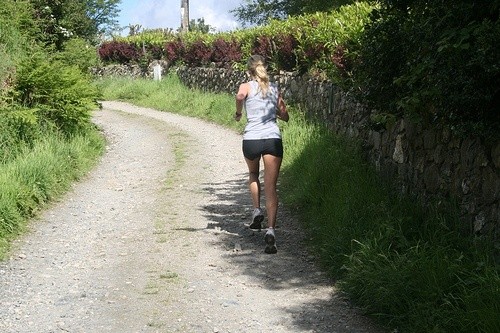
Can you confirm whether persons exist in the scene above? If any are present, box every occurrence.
[233,55,289,254]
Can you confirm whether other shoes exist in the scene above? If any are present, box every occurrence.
[263,226,278,253]
[248,207,264,230]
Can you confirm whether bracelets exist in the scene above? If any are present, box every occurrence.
[235,112,242,118]
